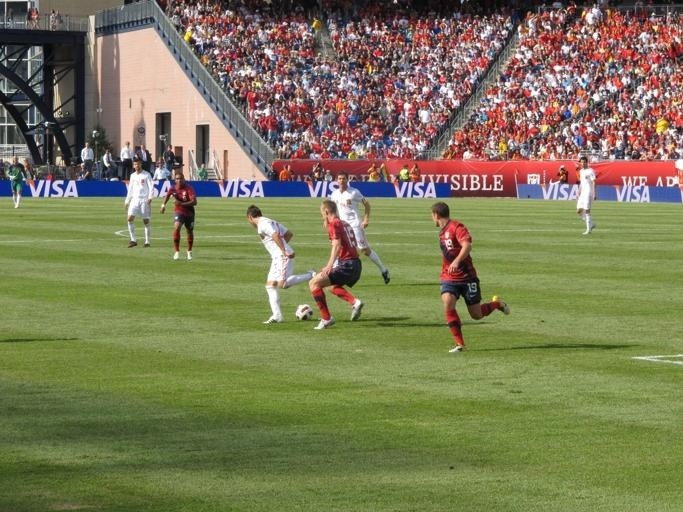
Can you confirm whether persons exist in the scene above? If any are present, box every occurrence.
[379,160,388,183]
[163,144,177,179]
[124,158,154,247]
[309,200,365,331]
[557,165,568,185]
[81,141,94,171]
[136,144,151,173]
[399,164,409,183]
[324,169,333,181]
[287,165,297,182]
[165,0,207,66]
[78,162,88,179]
[21,157,35,179]
[313,162,324,180]
[267,164,279,181]
[367,162,383,182]
[576,157,596,235]
[84,168,94,180]
[120,141,133,180]
[247,206,316,325]
[331,171,390,285]
[103,147,117,180]
[410,163,421,182]
[160,172,197,260]
[279,165,291,182]
[207,0,683,160]
[27,7,41,30]
[153,159,172,181]
[7,155,27,209]
[0,158,6,181]
[198,163,208,181]
[54,9,68,33]
[7,7,15,30]
[48,8,57,31]
[432,202,511,352]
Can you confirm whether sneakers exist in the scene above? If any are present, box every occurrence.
[144,243,149,247]
[127,241,137,249]
[448,345,464,353]
[309,271,317,278]
[262,315,284,324]
[492,296,510,315]
[382,268,390,285]
[174,252,179,260]
[187,251,193,261]
[582,231,592,235]
[350,299,364,321]
[312,316,335,330]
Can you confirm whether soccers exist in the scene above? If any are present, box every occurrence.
[296,303,314,321]
